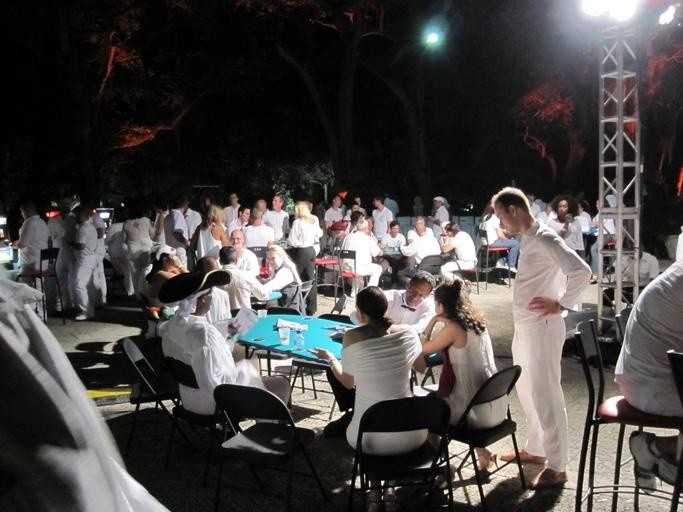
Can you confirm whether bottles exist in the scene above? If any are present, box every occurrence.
[294,329,305,353]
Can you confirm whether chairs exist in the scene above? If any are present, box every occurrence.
[407,255,445,288]
[160,357,238,488]
[287,312,354,411]
[313,241,341,296]
[214,383,332,511]
[334,250,368,299]
[17,248,66,324]
[342,395,459,511]
[665,349,683,512]
[616,306,636,350]
[120,338,185,465]
[478,229,513,288]
[262,306,305,376]
[575,319,680,511]
[264,279,314,314]
[435,365,526,507]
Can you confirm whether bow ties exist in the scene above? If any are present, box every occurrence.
[401,305,416,311]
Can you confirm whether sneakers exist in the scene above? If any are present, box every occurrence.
[76,313,92,319]
[505,265,517,273]
[495,260,510,269]
[629,431,660,493]
[643,431,683,486]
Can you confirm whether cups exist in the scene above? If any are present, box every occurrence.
[279,328,290,346]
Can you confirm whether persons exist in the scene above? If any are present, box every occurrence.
[230,229,260,272]
[572,200,592,262]
[379,220,406,247]
[348,211,364,235]
[341,220,384,298]
[48,197,69,249]
[158,267,293,421]
[162,197,189,254]
[383,271,436,334]
[226,206,251,242]
[189,203,228,260]
[612,258,683,497]
[320,220,347,271]
[344,201,366,222]
[241,207,273,249]
[121,202,159,304]
[372,192,394,244]
[490,187,593,491]
[407,215,433,248]
[441,221,478,296]
[261,191,288,243]
[62,206,98,322]
[92,214,108,308]
[426,195,449,243]
[222,192,242,225]
[397,223,444,287]
[102,209,128,274]
[589,198,616,285]
[215,245,270,310]
[478,200,520,274]
[260,244,306,318]
[288,201,323,316]
[256,198,269,217]
[4,202,50,324]
[322,194,344,232]
[305,284,428,512]
[547,194,585,259]
[420,278,508,489]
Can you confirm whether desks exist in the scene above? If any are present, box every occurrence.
[217,314,403,367]
[379,243,408,291]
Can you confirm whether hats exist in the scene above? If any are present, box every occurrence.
[434,197,445,203]
[159,269,232,303]
[152,242,176,260]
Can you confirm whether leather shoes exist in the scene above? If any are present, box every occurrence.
[529,468,567,487]
[501,447,547,466]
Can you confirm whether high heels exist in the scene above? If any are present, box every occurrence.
[477,454,500,471]
[364,491,380,512]
[436,465,456,489]
[383,488,396,512]
